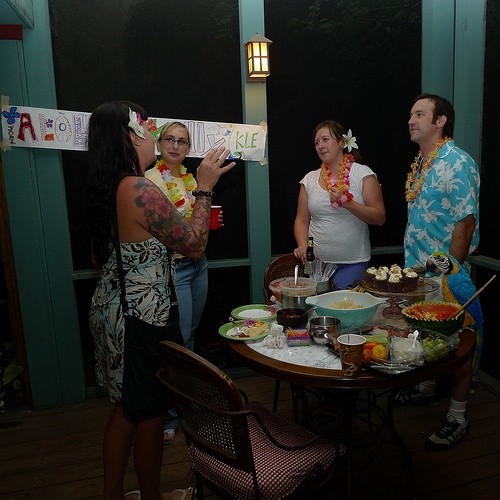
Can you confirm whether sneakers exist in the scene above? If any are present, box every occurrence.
[428,415,470,449]
[393,382,438,404]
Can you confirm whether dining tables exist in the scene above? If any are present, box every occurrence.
[227,303,478,443]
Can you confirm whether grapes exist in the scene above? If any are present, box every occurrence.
[422,338,448,362]
[386,326,409,338]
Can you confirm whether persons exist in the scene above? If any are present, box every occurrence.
[403,92,481,301]
[86,99,236,500]
[145,122,209,444]
[293,120,386,290]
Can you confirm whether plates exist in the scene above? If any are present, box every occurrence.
[218,319,271,340]
[328,317,458,372]
[232,305,279,322]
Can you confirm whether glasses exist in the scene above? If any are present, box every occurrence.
[160,137,191,146]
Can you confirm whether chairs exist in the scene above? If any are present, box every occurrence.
[155,339,346,500]
[264,252,311,420]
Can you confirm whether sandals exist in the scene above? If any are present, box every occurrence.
[163,429,175,444]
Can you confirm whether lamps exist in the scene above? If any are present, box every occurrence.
[244,32,273,83]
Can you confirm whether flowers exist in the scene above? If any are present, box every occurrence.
[128,108,146,141]
[343,129,358,153]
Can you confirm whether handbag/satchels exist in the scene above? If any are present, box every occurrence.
[121,314,187,414]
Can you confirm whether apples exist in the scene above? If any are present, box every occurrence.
[372,344,387,363]
[362,343,373,361]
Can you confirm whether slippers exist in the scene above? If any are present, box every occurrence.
[174,487,193,500]
[124,490,142,500]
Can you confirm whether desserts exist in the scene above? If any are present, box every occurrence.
[366,263,419,293]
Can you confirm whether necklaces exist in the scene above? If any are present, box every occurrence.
[320,154,354,194]
[155,160,196,222]
[404,136,454,202]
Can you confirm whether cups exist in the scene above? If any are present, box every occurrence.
[336,334,366,377]
[209,206,221,229]
[310,278,330,292]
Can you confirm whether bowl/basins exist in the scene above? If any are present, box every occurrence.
[309,315,340,345]
[306,289,388,326]
[268,278,287,302]
[277,308,308,329]
[280,277,317,309]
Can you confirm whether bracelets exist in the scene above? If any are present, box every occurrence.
[330,193,354,209]
[192,190,216,198]
